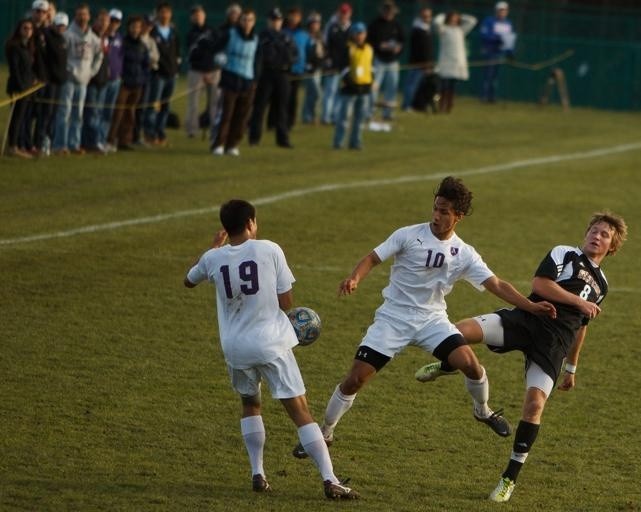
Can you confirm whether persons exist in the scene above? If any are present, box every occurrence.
[398,0,519,114]
[294,175,557,459]
[185,0,402,157]
[414,211,627,504]
[183,199,360,499]
[1,0,184,158]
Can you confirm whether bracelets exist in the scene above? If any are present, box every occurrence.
[563,360,578,376]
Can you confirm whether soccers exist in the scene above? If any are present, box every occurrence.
[286,307,321,345]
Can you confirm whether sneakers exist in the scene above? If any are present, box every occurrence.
[253,361,514,503]
